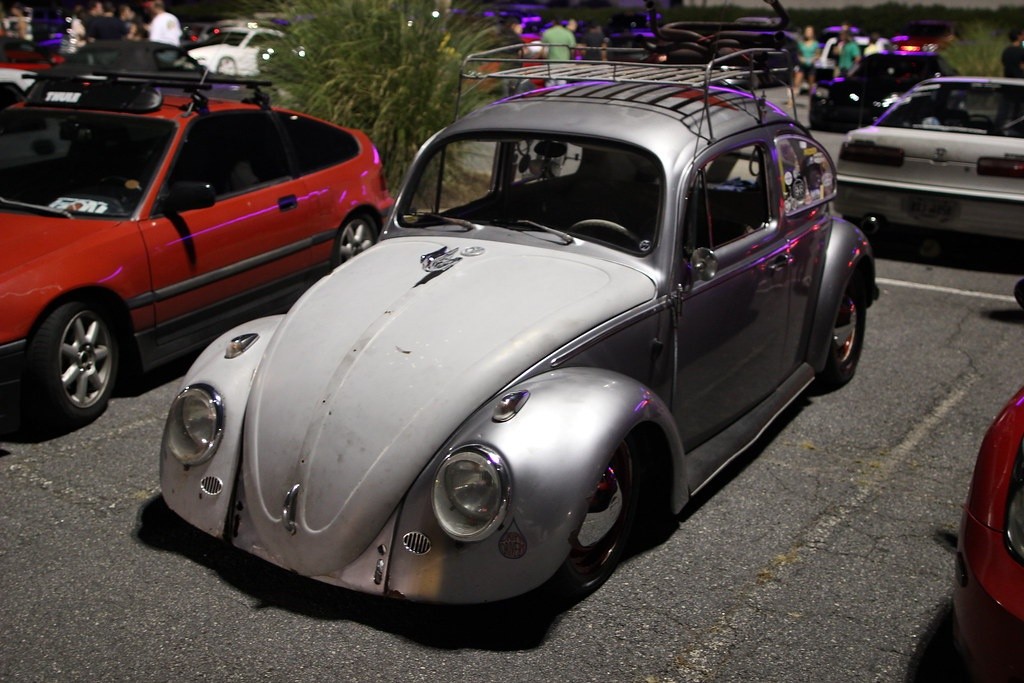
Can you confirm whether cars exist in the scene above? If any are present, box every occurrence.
[605,12,665,57]
[888,20,956,54]
[158,81,877,610]
[445,8,543,35]
[516,32,548,89]
[809,50,964,131]
[817,26,863,52]
[0,13,297,107]
[950,386,1024,683]
[0,40,400,436]
[834,76,1024,240]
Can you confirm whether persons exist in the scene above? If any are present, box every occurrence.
[0,0,183,69]
[492,12,608,101]
[786,23,892,112]
[994,25,1024,130]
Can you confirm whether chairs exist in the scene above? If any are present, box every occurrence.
[548,146,655,251]
